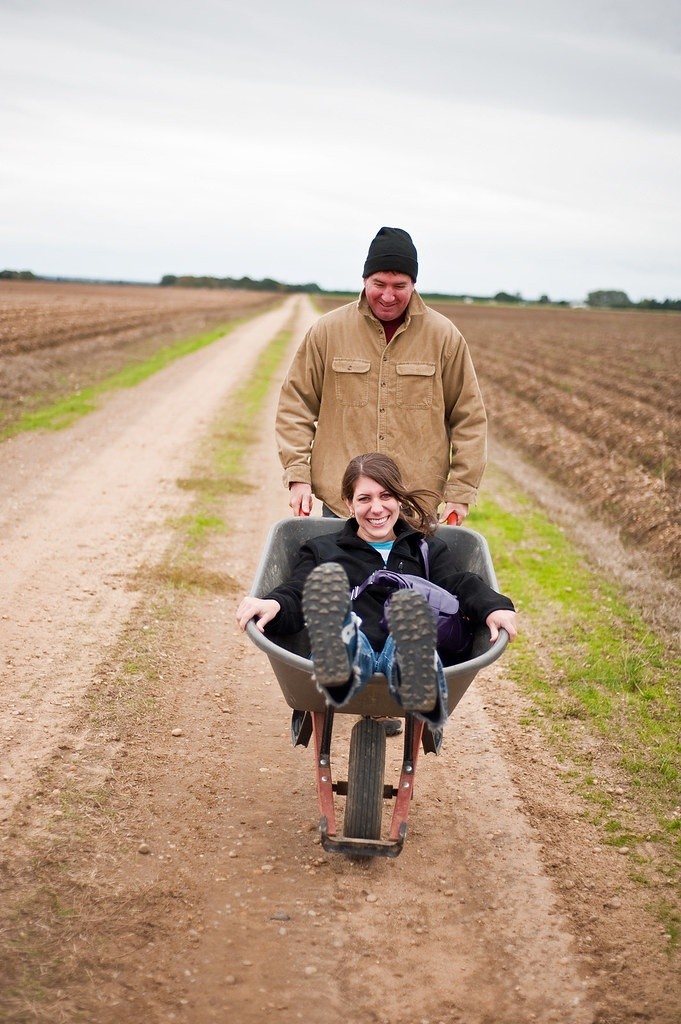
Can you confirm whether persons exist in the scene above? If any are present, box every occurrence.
[275,223,489,736]
[232,452,519,725]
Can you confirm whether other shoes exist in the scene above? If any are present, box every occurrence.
[386,587,437,712]
[361,716,402,737]
[302,561,350,685]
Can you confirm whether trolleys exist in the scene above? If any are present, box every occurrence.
[243,499,512,866]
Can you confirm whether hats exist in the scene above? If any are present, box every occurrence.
[362,227,418,282]
